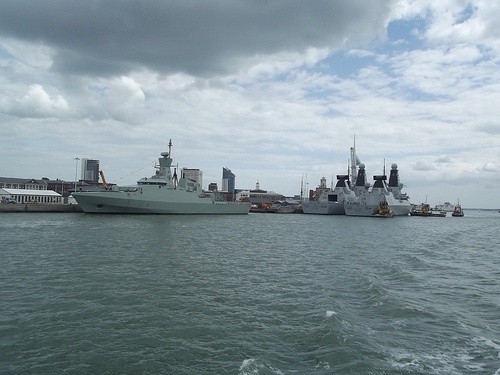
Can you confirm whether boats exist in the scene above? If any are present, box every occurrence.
[300,133,465,218]
[71,138,253,215]
